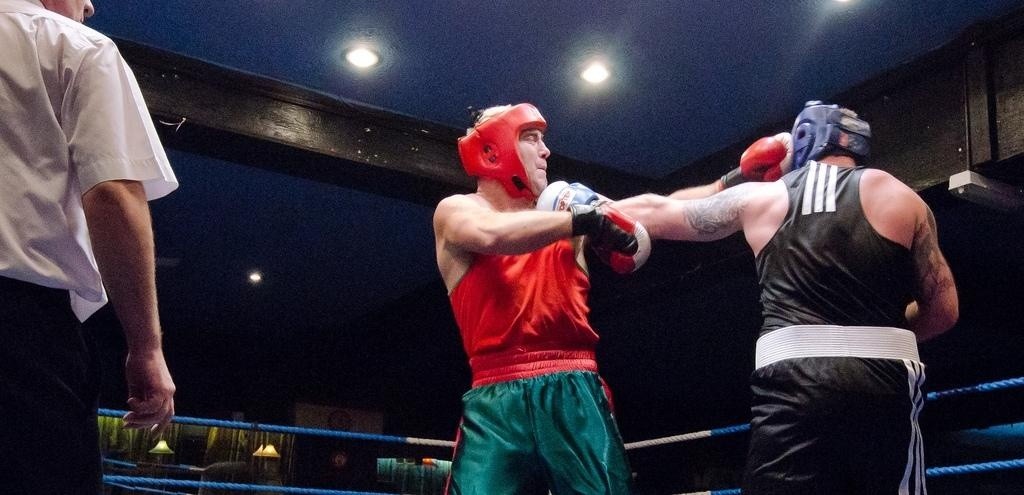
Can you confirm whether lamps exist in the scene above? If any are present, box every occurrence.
[948,46,1024,210]
[253,431,281,458]
[147,431,175,455]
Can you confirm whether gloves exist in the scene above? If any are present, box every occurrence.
[720,131,794,190]
[567,198,651,275]
[536,180,599,212]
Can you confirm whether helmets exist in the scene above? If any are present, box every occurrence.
[457,101,548,198]
[792,100,872,169]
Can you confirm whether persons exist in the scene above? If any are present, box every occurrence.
[0,0,182,493]
[431,101,797,493]
[534,96,963,495]
